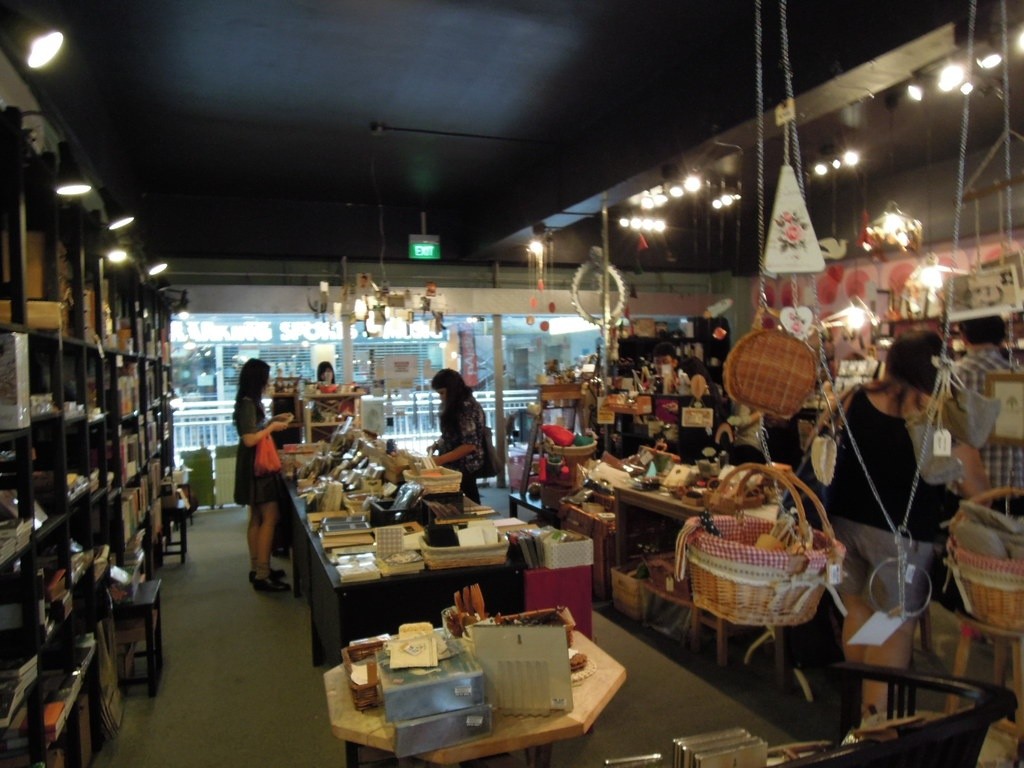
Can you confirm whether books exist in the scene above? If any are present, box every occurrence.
[0,280,170,768]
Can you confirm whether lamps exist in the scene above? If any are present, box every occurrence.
[305,158,445,342]
[863,94,923,249]
[2,14,166,278]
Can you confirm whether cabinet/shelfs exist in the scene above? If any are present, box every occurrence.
[608,390,723,468]
[0,107,173,768]
[273,391,365,445]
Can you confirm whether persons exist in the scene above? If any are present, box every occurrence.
[950,314,1024,522]
[425,369,485,507]
[233,359,290,594]
[818,330,995,715]
[650,342,767,466]
[313,361,341,442]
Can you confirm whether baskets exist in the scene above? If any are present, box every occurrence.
[946,486,1024,629]
[686,463,841,628]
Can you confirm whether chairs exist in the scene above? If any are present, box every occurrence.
[764,657,1017,767]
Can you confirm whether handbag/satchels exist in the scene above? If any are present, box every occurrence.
[253,430,281,477]
[475,427,502,478]
[777,385,855,532]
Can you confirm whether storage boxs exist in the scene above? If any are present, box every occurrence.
[371,626,492,760]
[530,527,595,570]
[0,695,92,768]
[611,566,654,621]
[1,229,109,431]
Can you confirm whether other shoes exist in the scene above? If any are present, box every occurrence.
[248,569,290,592]
[859,709,917,730]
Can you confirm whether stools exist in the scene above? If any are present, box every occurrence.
[691,603,785,666]
[950,614,1024,753]
[162,514,187,563]
[113,578,164,698]
[178,483,193,524]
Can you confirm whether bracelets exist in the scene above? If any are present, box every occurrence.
[434,441,439,449]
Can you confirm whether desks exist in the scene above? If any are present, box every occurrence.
[277,449,528,671]
[507,481,710,600]
[324,631,628,768]
